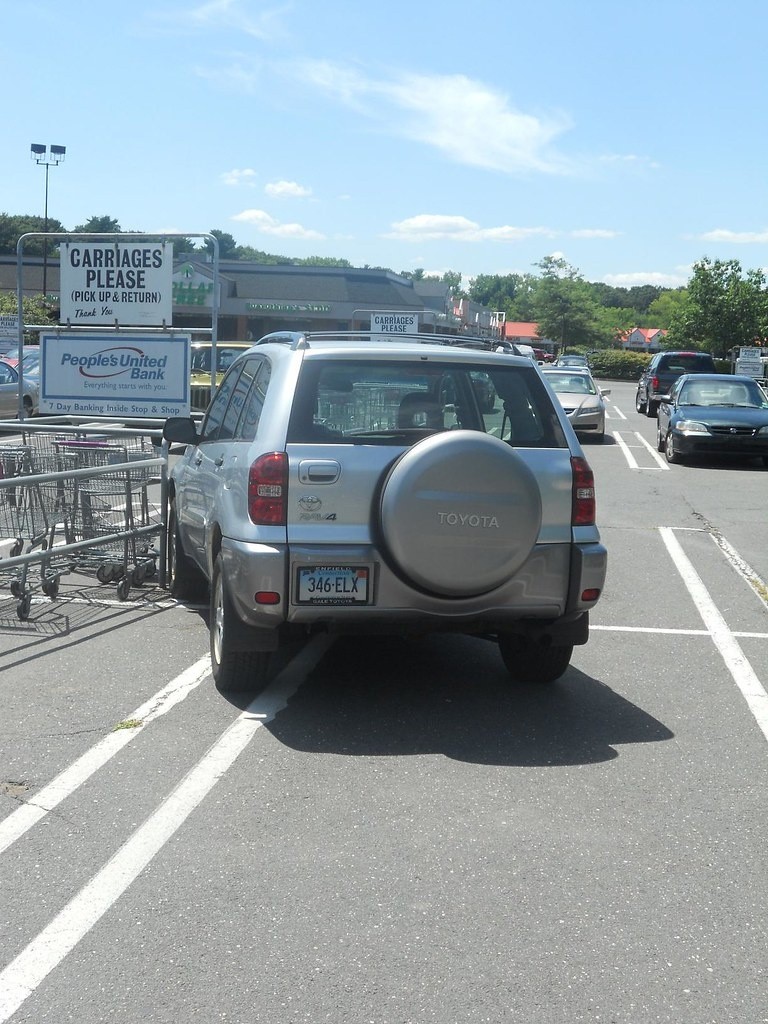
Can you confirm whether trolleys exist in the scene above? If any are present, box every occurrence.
[0,430,163,620]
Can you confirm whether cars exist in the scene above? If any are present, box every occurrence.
[0,344,43,420]
[540,367,611,444]
[189,340,260,412]
[533,347,589,366]
[656,373,768,463]
[636,351,718,418]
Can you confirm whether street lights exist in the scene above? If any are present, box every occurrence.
[31,143,66,297]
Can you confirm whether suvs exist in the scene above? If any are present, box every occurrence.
[163,331,608,693]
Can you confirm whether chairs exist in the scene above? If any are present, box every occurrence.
[311,423,344,443]
[569,378,584,392]
[661,363,670,371]
[729,387,746,404]
[398,392,445,428]
[685,390,702,403]
[684,362,695,370]
